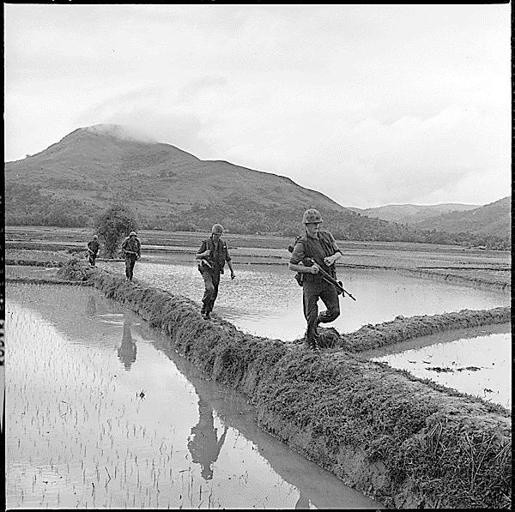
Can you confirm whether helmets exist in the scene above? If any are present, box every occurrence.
[302,209,323,224]
[129,232,138,238]
[211,224,223,235]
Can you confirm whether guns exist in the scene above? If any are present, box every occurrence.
[83,247,94,255]
[287,244,358,301]
[198,255,215,270]
[115,249,138,257]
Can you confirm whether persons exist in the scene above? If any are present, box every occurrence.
[87,234,102,265]
[195,224,236,321]
[120,231,141,280]
[116,319,138,373]
[287,208,342,355]
[187,393,230,483]
[84,293,100,317]
